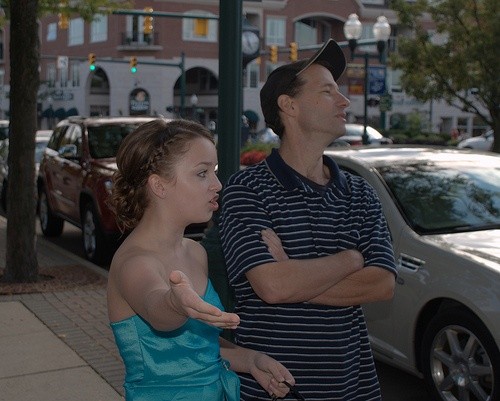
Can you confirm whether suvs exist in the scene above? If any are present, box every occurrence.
[36,115,219,263]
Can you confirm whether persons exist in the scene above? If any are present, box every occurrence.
[105,119,296,401]
[219,38,398,401]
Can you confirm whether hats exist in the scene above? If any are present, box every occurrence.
[260,38,347,129]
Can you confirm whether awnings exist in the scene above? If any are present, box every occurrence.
[41,108,54,117]
[52,108,65,117]
[64,108,79,117]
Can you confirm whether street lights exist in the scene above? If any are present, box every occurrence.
[190,95,198,123]
[343,13,391,145]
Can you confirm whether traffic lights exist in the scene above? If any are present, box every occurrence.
[290,41,298,62]
[142,8,153,35]
[88,53,96,72]
[58,2,67,30]
[129,58,137,74]
[268,45,278,64]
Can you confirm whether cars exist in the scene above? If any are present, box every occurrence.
[239,129,500,401]
[328,124,392,150]
[0,120,54,215]
